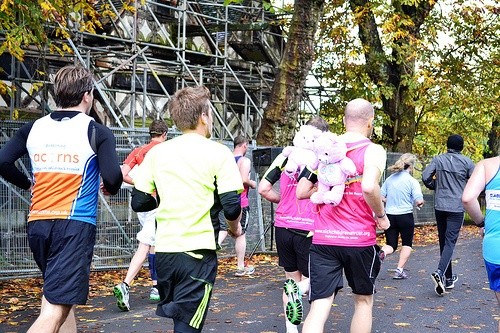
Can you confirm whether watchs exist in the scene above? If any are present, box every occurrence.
[301,97,391,332]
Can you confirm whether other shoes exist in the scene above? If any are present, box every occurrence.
[234,265,255,276]
[282,278,304,326]
[445,273,459,289]
[392,269,407,279]
[379,249,386,263]
[431,271,445,297]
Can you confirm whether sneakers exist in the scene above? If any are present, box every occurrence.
[149,287,160,300]
[113,283,131,311]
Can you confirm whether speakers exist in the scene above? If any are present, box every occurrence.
[253,148,288,167]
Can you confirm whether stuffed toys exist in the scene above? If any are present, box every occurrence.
[281,124,322,177]
[310,130,357,207]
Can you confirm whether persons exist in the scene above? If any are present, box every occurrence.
[131,84,244,333]
[377,153,424,279]
[462,126,500,305]
[421,134,475,296]
[257,117,330,333]
[0,64,123,333]
[113,119,168,311]
[217,134,257,276]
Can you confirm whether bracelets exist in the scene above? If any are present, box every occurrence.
[378,211,385,219]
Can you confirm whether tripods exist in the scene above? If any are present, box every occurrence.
[249,167,276,262]
